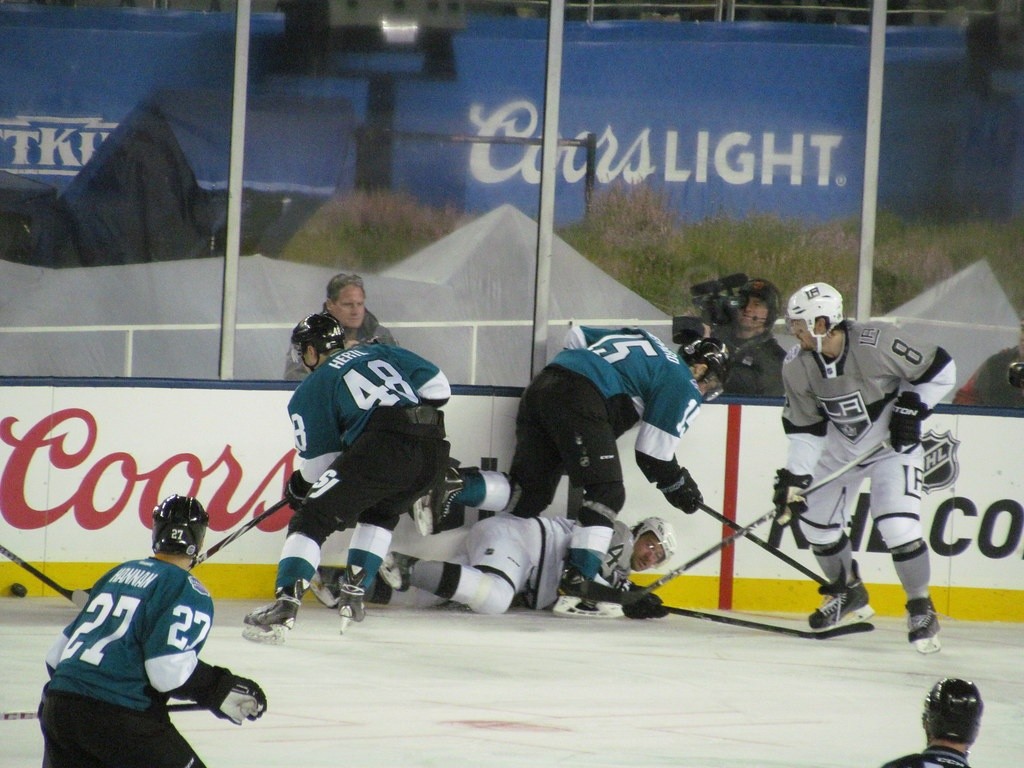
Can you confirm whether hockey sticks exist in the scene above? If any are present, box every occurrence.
[0,700,256,722]
[188,497,289,571]
[581,438,893,605]
[0,546,93,609]
[691,498,830,587]
[659,605,875,640]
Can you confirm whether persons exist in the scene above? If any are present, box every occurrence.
[770,283,956,656]
[952,319,1024,409]
[880,678,983,768]
[285,270,396,381]
[37,494,267,768]
[679,278,788,397]
[308,515,676,618]
[408,321,732,617]
[243,314,450,645]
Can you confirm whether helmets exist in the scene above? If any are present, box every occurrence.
[923,678,984,744]
[679,338,734,385]
[785,282,843,335]
[740,278,781,326]
[152,494,208,555]
[629,516,676,568]
[291,314,345,353]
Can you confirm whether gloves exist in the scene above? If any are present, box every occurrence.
[284,469,314,511]
[888,392,933,452]
[769,469,813,528]
[624,590,668,619]
[656,467,702,514]
[198,666,268,725]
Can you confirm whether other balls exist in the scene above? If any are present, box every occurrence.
[11,583,27,597]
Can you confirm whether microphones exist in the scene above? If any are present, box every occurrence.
[689,273,748,295]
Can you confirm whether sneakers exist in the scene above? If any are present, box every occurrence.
[338,564,366,635]
[553,567,625,620]
[242,578,311,644]
[310,566,347,608]
[378,552,420,592]
[808,559,875,632]
[905,598,941,654]
[413,467,464,537]
